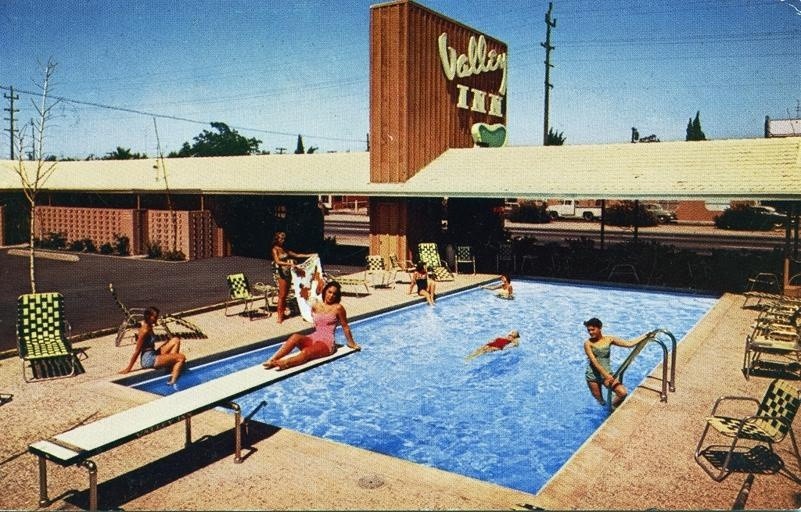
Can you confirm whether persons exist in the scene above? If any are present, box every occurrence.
[272,229,318,325]
[463,327,521,362]
[407,260,438,308]
[116,305,187,389]
[582,317,658,408]
[480,274,514,300]
[261,280,363,371]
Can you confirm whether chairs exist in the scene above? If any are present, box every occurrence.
[695,273,801,481]
[16,292,74,383]
[109,283,203,347]
[497,241,517,274]
[225,272,270,321]
[322,243,476,298]
[272,260,304,317]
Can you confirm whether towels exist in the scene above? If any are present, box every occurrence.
[291,253,326,323]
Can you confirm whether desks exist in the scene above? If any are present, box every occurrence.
[251,285,276,314]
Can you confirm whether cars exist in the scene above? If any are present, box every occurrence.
[505,198,602,222]
[749,206,795,228]
[640,203,676,224]
